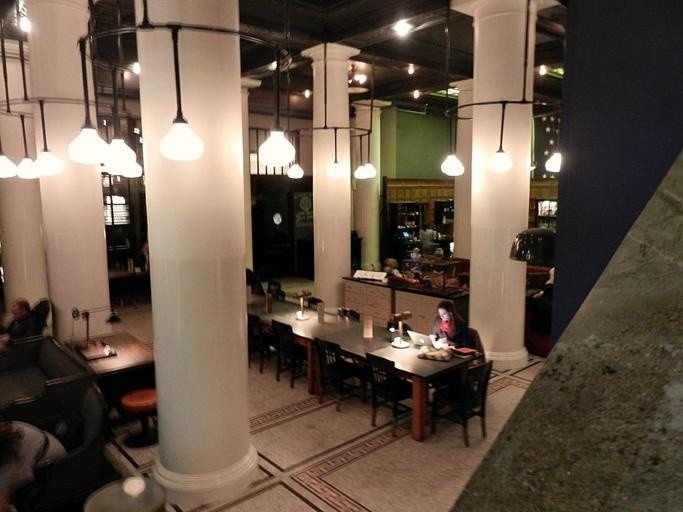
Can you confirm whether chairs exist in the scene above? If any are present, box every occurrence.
[5,379,108,512]
[0,298,51,345]
[246,279,491,447]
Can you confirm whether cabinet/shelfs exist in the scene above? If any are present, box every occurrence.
[390,199,427,239]
[431,200,454,236]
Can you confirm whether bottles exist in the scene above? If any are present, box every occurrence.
[388,327,399,342]
[442,206,454,223]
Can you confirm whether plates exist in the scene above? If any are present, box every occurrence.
[391,341,410,349]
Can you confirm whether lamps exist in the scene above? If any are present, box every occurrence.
[0,0,531,182]
[506,181,555,270]
[70,307,122,350]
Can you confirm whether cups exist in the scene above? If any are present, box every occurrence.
[393,337,401,345]
[245,285,252,304]
[265,294,272,314]
[317,301,325,324]
[127,258,133,273]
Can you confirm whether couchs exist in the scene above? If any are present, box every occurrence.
[0,333,96,420]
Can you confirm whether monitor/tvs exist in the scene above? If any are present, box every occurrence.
[402,231,410,237]
[536,199,558,218]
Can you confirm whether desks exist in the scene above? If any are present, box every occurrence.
[71,332,155,428]
[402,256,461,286]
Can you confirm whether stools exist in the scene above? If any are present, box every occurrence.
[119,389,157,449]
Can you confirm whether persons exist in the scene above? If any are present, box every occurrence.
[0,421,72,512]
[0,297,36,352]
[137,243,149,272]
[432,302,469,348]
[418,224,433,257]
[384,258,402,279]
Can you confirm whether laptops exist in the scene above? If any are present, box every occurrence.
[407,330,444,350]
